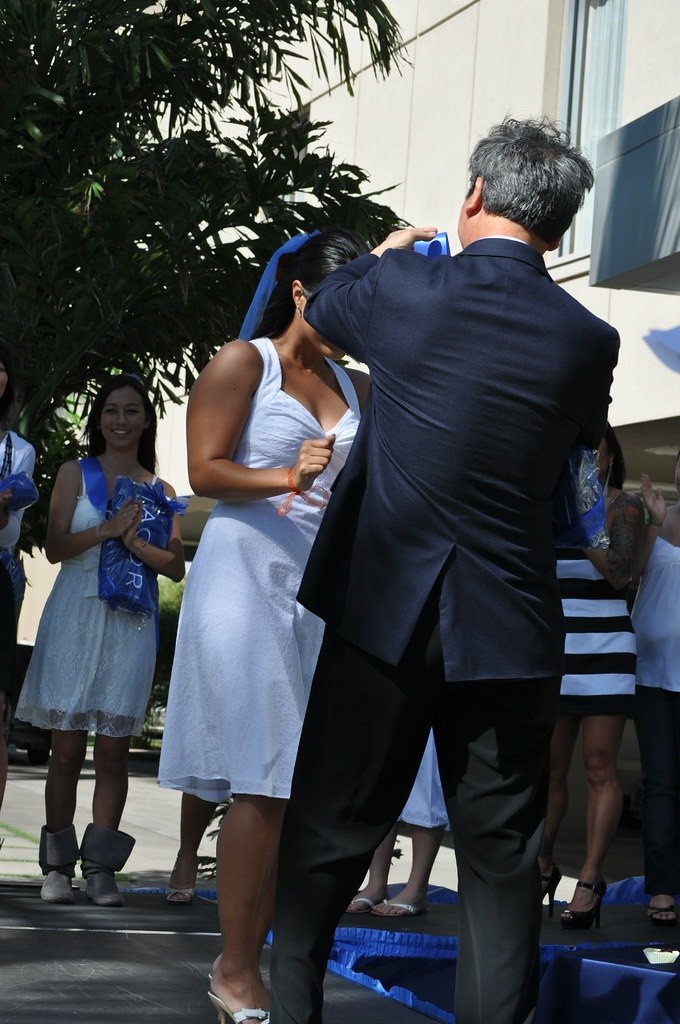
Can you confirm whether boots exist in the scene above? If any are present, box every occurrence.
[38,823,79,903]
[79,823,136,906]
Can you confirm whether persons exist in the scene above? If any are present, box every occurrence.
[159,228,371,1024]
[14,374,186,906]
[268,112,621,1024]
[0,337,38,813]
[164,419,680,931]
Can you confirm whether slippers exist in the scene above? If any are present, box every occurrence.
[371,898,429,917]
[345,897,390,913]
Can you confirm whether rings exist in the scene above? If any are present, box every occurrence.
[123,513,126,518]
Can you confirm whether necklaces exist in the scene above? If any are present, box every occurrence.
[100,455,141,480]
[0,432,12,480]
[607,492,620,506]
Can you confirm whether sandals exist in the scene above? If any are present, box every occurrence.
[646,893,678,927]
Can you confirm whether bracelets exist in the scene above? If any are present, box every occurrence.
[648,519,663,527]
[133,538,147,556]
[96,519,107,543]
[277,466,330,517]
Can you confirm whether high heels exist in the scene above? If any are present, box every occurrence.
[207,991,270,1024]
[206,973,226,1022]
[538,860,561,919]
[560,875,607,930]
[164,856,199,904]
